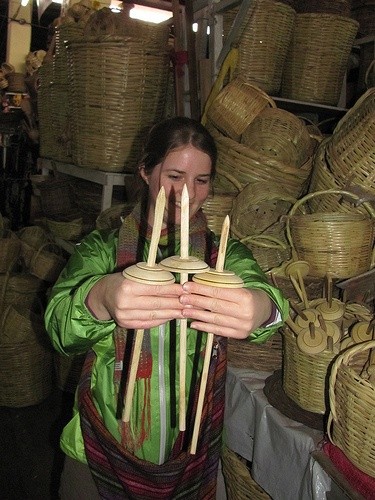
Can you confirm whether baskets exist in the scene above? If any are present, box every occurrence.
[0,63,28,94]
[222,0,296,99]
[279,13,359,106]
[201,76,375,277]
[279,297,375,414]
[0,175,138,408]
[32,24,170,175]
[220,445,275,500]
[227,275,341,372]
[327,341,375,478]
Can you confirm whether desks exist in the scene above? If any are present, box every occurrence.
[36,158,135,213]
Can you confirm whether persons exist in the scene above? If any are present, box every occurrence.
[44,116,289,500]
